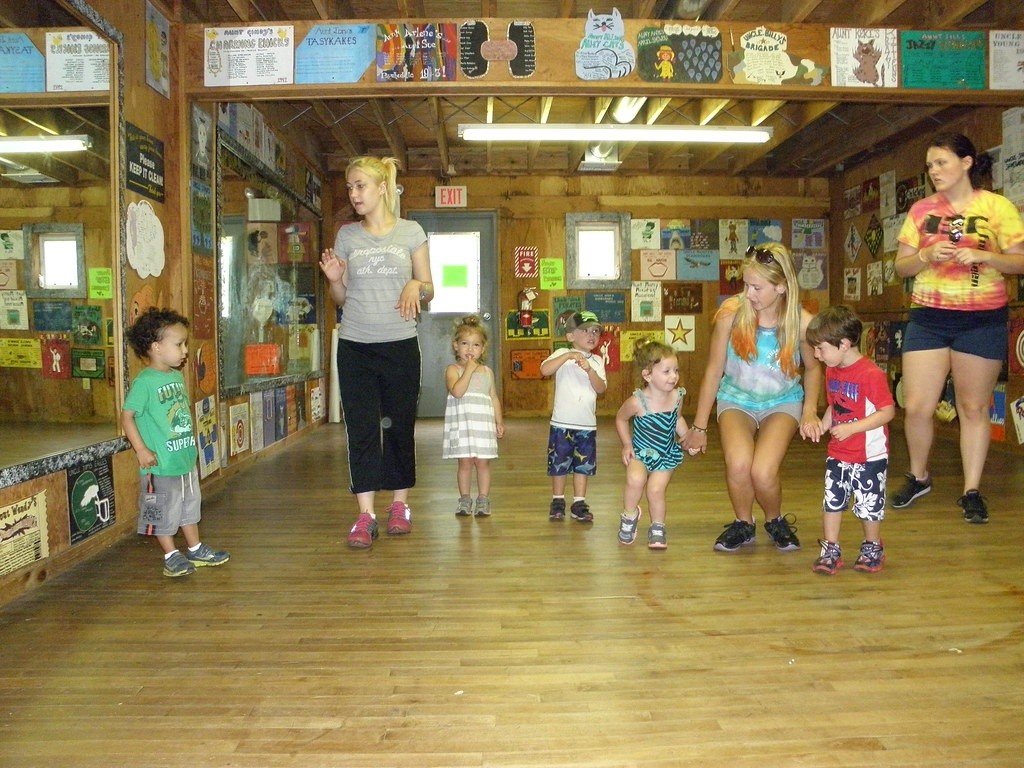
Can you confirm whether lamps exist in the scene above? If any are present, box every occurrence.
[0,134,94,153]
[457,123,774,144]
[245,185,280,222]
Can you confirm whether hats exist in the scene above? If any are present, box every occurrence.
[566,310,602,333]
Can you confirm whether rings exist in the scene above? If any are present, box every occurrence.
[937,255,940,259]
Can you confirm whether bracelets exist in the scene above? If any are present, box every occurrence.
[919,246,928,263]
[418,281,425,300]
[691,424,708,434]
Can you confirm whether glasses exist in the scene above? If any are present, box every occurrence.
[745,245,783,272]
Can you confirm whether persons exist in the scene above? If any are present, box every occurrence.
[539,310,608,521]
[50,347,61,373]
[615,336,701,549]
[441,314,504,517]
[119,307,231,577]
[802,305,896,575]
[887,131,1024,525]
[678,241,824,555]
[318,155,434,548]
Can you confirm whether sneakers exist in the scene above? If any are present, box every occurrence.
[891,471,931,509]
[164,551,195,576]
[618,505,641,544]
[185,542,231,568]
[958,488,989,523]
[813,538,843,575]
[385,501,412,535]
[456,496,472,515]
[647,522,667,548]
[714,517,756,552]
[853,538,885,573]
[764,513,801,551]
[570,500,593,520]
[550,498,565,518]
[348,509,380,549]
[475,496,491,516]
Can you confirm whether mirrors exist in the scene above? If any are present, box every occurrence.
[0,0,132,490]
[214,125,327,404]
